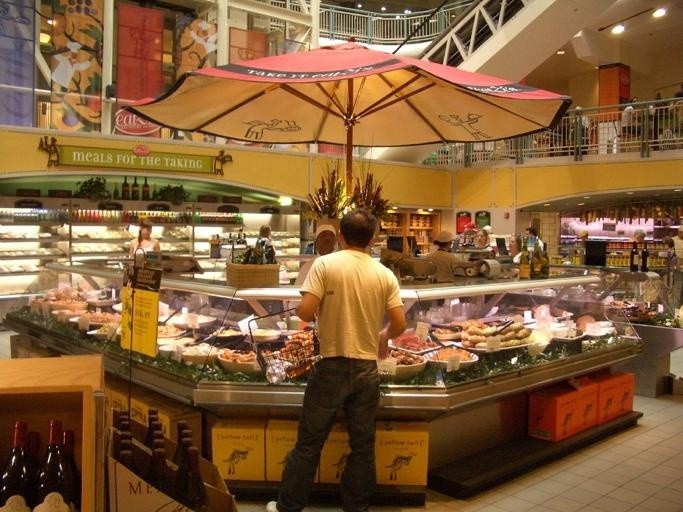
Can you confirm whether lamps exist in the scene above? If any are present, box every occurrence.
[598,7,666,34]
[275,196,290,204]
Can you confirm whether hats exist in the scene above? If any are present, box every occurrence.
[316,223,337,239]
[436,230,455,244]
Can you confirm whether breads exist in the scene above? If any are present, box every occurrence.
[461,324,532,349]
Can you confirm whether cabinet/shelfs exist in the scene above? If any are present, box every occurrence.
[560,235,673,271]
[599,270,683,329]
[1,261,646,412]
[369,209,441,257]
[0,209,301,300]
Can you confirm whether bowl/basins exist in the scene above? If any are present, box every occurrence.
[246,329,281,345]
[282,315,311,330]
[381,354,427,380]
[421,347,479,372]
[216,348,257,375]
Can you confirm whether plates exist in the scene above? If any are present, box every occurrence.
[30,289,217,367]
[216,330,243,340]
[517,312,614,342]
[385,338,443,355]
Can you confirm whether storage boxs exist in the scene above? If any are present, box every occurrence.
[528,376,598,443]
[594,367,634,426]
[10,335,428,494]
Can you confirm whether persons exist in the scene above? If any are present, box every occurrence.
[266,209,407,512]
[128,220,162,268]
[255,224,276,263]
[288,224,337,316]
[426,224,683,309]
[550,83,683,157]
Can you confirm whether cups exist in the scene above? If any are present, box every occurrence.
[550,255,561,265]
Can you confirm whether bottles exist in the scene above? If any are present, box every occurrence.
[629,241,639,272]
[640,241,649,273]
[111,175,158,201]
[0,207,243,225]
[571,253,583,265]
[284,354,323,379]
[518,235,549,281]
[0,418,80,512]
[115,406,208,512]
[208,233,221,258]
[604,242,670,267]
[227,232,247,245]
[410,214,431,228]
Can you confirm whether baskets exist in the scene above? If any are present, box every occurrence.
[227,261,280,288]
[248,309,321,386]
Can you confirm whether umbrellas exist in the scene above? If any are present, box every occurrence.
[120,37,574,210]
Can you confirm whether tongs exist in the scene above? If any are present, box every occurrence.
[426,321,462,348]
[482,318,514,336]
